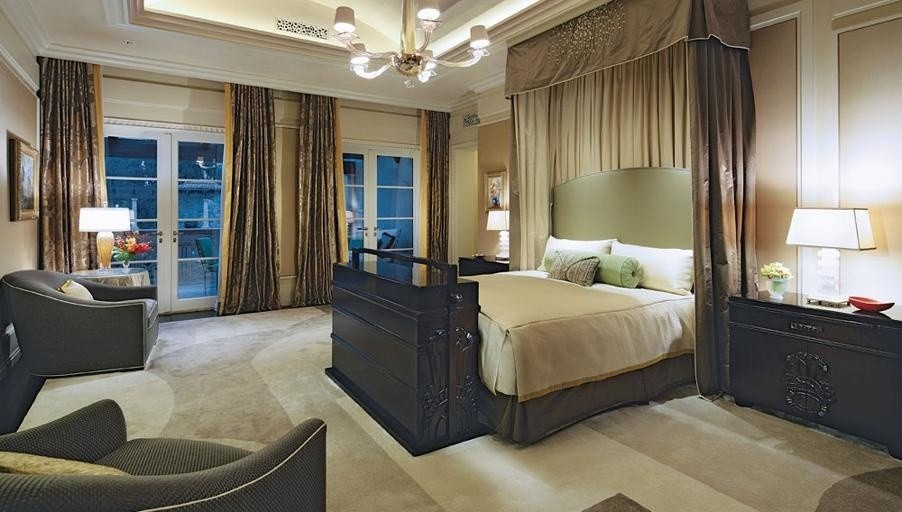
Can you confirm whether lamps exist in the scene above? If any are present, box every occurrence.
[78,206,131,271]
[785,206,876,308]
[486,209,509,261]
[331,0,491,88]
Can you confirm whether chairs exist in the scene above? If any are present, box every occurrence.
[197,238,223,296]
[1,402,327,511]
[2,270,158,377]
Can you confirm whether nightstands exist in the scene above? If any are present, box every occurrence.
[725,293,901,459]
[459,256,508,276]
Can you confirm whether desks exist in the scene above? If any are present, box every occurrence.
[70,266,150,288]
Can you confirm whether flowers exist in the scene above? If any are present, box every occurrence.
[112,233,153,265]
[760,262,790,293]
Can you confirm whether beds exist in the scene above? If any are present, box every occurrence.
[456,269,694,444]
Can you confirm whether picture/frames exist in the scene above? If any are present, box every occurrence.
[10,136,41,221]
[483,171,507,213]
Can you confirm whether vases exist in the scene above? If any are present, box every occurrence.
[765,278,787,299]
[123,257,131,274]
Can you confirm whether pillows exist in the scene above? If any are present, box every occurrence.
[537,236,695,298]
[0,449,129,478]
[60,278,94,301]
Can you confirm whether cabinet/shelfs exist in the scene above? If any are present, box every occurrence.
[139,230,218,286]
